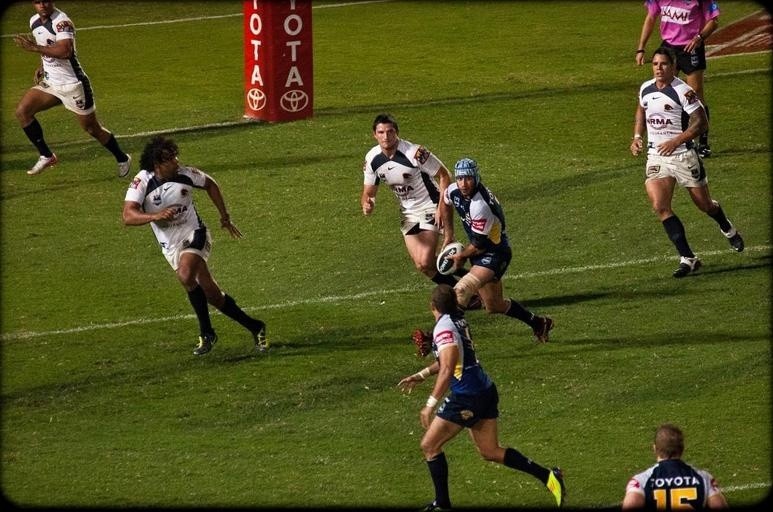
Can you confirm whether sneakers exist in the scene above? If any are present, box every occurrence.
[674,255,700,278]
[117,153,132,177]
[193,333,218,355]
[413,329,431,357]
[720,218,744,252]
[534,316,554,343]
[423,500,444,511]
[699,146,712,158]
[254,319,272,353]
[545,467,567,507]
[27,152,57,175]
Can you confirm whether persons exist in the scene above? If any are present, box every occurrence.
[621,424,727,510]
[360,116,483,310]
[630,46,744,279]
[412,158,553,358]
[398,284,565,512]
[13,1,132,178]
[121,135,268,356]
[636,0,721,158]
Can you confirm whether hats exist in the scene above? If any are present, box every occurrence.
[455,157,481,188]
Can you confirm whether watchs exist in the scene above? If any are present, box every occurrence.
[696,33,705,41]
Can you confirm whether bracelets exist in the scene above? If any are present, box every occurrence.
[634,135,642,139]
[426,395,438,409]
[221,214,230,224]
[419,366,431,380]
[637,50,645,53]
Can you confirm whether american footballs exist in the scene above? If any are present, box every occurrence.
[436,242,467,276]
